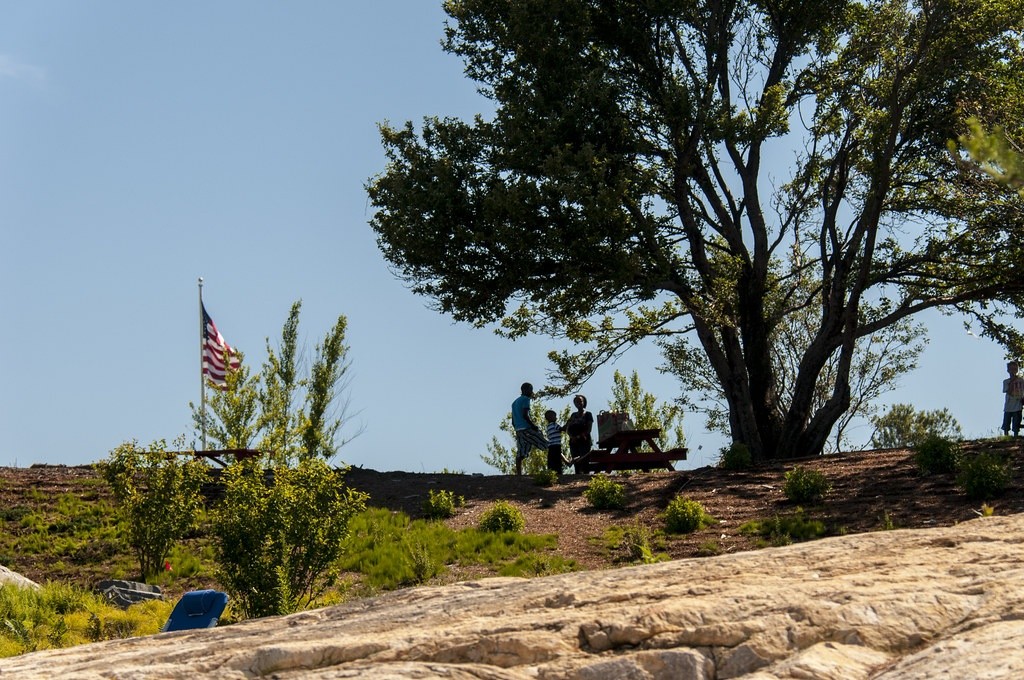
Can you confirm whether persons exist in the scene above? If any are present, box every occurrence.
[511,381,581,477]
[566,393,593,474]
[1001,361,1024,437]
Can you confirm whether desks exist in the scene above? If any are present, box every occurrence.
[594,429,676,474]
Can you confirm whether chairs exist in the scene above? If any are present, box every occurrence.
[162,590,227,632]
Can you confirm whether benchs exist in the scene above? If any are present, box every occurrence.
[667,448,689,460]
[574,450,610,466]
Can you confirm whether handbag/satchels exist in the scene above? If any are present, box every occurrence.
[598,408,634,441]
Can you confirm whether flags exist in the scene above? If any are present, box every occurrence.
[202,302,240,391]
